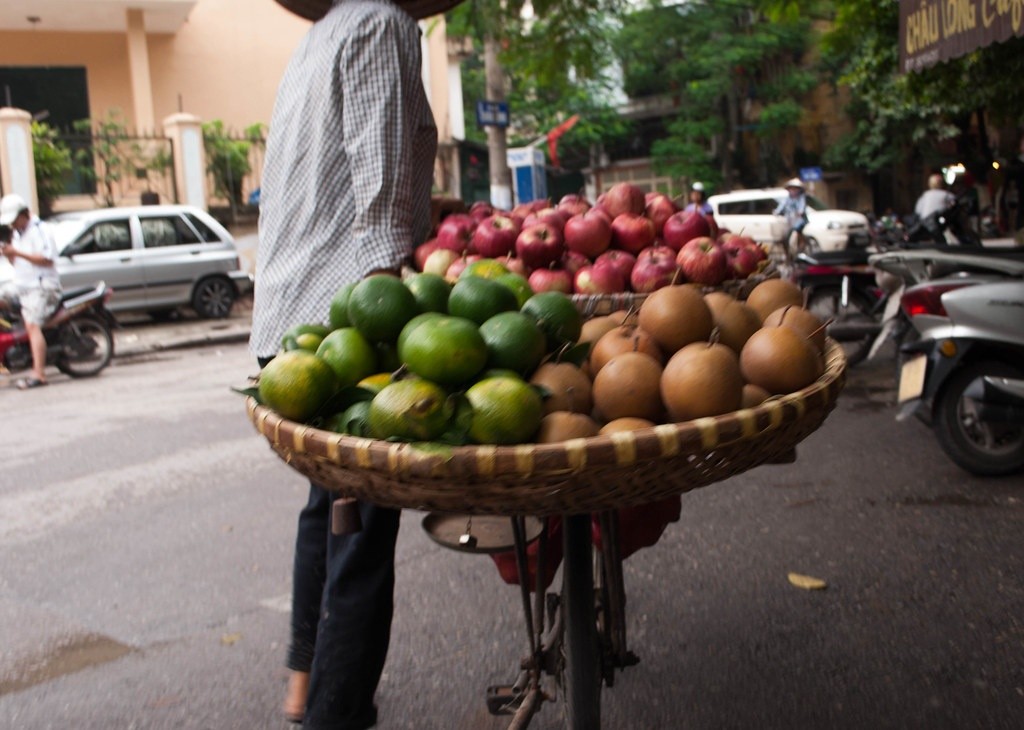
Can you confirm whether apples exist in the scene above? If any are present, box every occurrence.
[412,180,769,297]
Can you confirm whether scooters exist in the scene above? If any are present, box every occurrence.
[787,154,1024,479]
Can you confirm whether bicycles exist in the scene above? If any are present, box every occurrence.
[487,514,640,729]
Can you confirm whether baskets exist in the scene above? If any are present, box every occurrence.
[245,303,850,518]
[567,255,782,313]
[770,221,790,242]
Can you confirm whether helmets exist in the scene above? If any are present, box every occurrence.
[692,181,705,193]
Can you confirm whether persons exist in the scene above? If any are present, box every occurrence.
[684,181,713,218]
[773,178,808,265]
[915,174,958,219]
[0,193,65,390]
[246,0,471,730]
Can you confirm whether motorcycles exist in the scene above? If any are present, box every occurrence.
[0,282,118,381]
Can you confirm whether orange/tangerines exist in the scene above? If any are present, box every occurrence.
[257,258,583,450]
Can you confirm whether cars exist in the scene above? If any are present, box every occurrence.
[41,205,254,332]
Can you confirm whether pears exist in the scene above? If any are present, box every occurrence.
[526,264,833,444]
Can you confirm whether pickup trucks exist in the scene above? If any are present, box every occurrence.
[699,191,872,255]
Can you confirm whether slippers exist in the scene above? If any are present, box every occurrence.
[15,377,48,389]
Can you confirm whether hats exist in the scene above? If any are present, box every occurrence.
[787,177,804,191]
[0,194,27,226]
